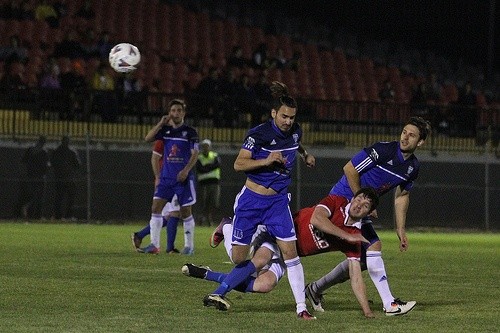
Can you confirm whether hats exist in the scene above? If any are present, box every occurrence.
[202,139,211,146]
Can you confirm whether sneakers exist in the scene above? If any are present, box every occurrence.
[202,294,231,311]
[181,247,194,254]
[182,263,212,279]
[295,304,317,320]
[136,243,161,255]
[210,217,232,248]
[305,282,327,312]
[131,232,142,251]
[166,249,180,255]
[383,298,416,316]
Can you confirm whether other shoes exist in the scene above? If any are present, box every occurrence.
[21,206,27,218]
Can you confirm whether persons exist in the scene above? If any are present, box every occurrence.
[132,98,199,256]
[181,189,380,318]
[196,138,222,227]
[13,135,83,218]
[210,81,318,320]
[0,0,500,124]
[304,117,431,317]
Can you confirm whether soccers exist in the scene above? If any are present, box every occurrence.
[109,43,142,74]
